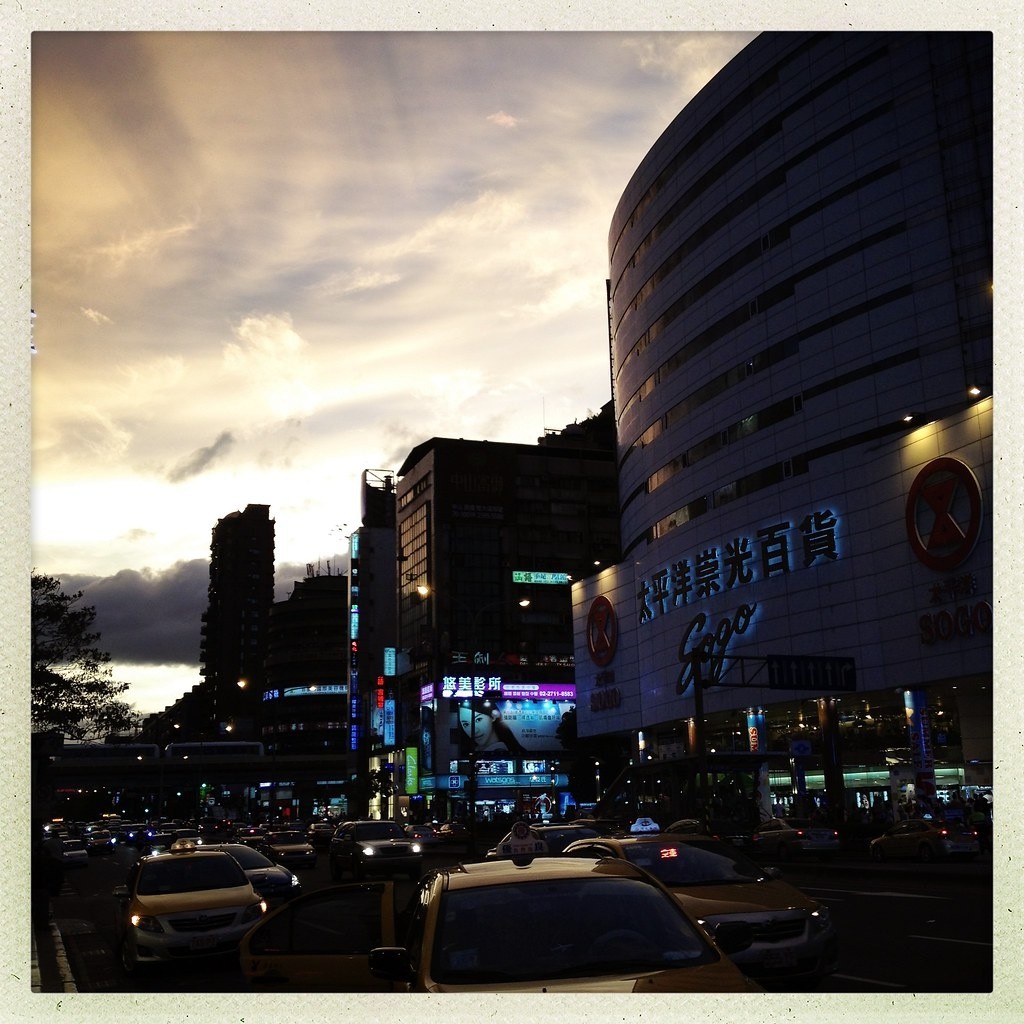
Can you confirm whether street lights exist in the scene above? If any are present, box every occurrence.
[137,754,189,814]
[414,585,532,819]
[237,679,317,820]
[173,723,233,816]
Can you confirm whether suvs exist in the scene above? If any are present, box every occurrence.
[330,821,424,882]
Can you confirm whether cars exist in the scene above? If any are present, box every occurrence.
[236,822,766,992]
[195,844,303,909]
[752,817,839,863]
[484,819,623,861]
[867,814,982,863]
[664,817,773,867]
[561,819,836,989]
[112,837,268,970]
[44,815,337,869]
[404,823,471,850]
